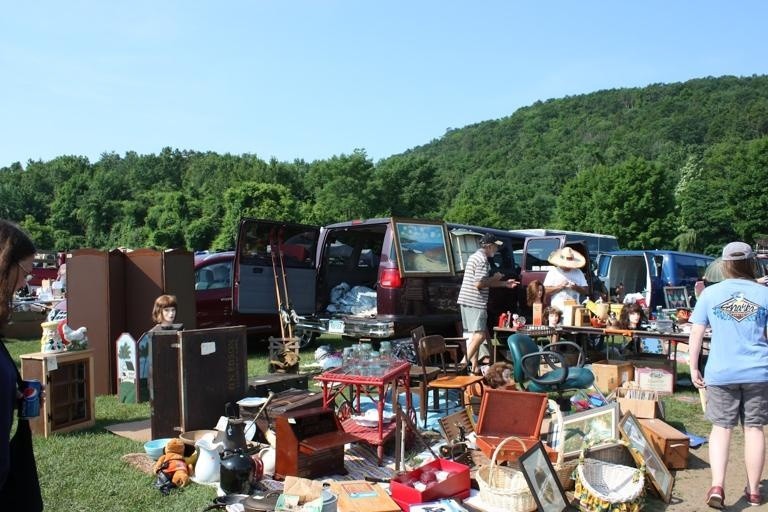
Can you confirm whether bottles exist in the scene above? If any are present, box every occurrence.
[498,311,526,331]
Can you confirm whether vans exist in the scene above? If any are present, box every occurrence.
[231,216,568,352]
[509,229,620,271]
[595,250,717,320]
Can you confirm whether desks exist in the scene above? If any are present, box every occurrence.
[561,325,711,371]
[493,324,563,363]
[313,355,417,467]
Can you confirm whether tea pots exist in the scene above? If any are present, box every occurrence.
[192,437,226,483]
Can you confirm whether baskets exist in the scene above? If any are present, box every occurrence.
[548,398,579,490]
[475,436,537,512]
[574,439,647,512]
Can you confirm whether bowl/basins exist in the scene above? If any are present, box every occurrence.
[179,428,220,445]
[143,438,174,459]
[350,414,380,428]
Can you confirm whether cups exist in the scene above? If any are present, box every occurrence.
[341,341,391,378]
[698,387,707,415]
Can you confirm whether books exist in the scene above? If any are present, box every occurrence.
[624,390,654,400]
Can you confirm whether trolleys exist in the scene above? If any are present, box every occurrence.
[268,339,300,374]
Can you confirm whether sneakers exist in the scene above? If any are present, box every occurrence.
[707,486,725,509]
[744,486,762,505]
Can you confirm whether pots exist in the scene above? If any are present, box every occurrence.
[203,483,337,512]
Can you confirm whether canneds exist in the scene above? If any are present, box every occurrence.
[20,380,42,421]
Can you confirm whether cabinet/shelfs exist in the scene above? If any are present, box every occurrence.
[20,349,96,441]
[147,325,248,458]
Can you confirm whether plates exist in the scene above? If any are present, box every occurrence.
[682,431,708,449]
[243,419,257,440]
[238,395,268,407]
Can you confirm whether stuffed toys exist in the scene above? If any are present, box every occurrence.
[154,438,198,487]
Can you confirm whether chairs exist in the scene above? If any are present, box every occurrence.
[411,325,471,409]
[507,333,594,416]
[418,335,484,428]
[208,266,228,289]
[195,270,213,290]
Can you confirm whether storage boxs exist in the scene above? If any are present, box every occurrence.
[592,360,633,395]
[637,418,690,469]
[248,373,308,398]
[633,365,673,395]
[475,389,558,462]
[606,387,665,418]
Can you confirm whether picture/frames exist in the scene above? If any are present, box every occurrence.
[519,441,571,512]
[617,410,674,504]
[547,403,620,462]
[391,216,456,278]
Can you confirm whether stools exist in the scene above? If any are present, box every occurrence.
[392,366,441,420]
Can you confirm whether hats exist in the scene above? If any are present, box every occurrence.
[480,233,504,246]
[722,242,753,260]
[549,247,586,268]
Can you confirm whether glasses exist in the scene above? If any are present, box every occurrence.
[17,262,37,283]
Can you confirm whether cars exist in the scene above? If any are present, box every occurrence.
[193,251,261,325]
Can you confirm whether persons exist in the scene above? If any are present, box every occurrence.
[620,303,644,329]
[541,246,590,326]
[152,295,178,326]
[0,217,47,511]
[483,362,513,388]
[689,241,768,510]
[526,279,545,306]
[542,306,563,327]
[456,232,521,376]
